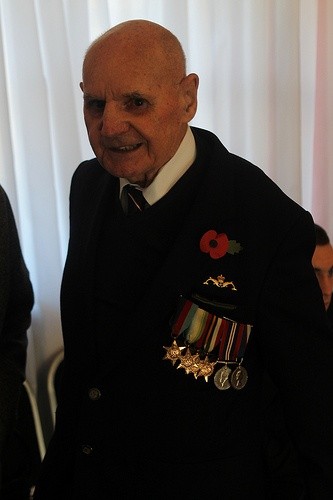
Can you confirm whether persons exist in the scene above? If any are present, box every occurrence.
[310,222,332,312]
[0,184,44,500]
[22,20,332,500]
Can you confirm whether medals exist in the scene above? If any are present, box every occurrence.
[163,337,248,390]
